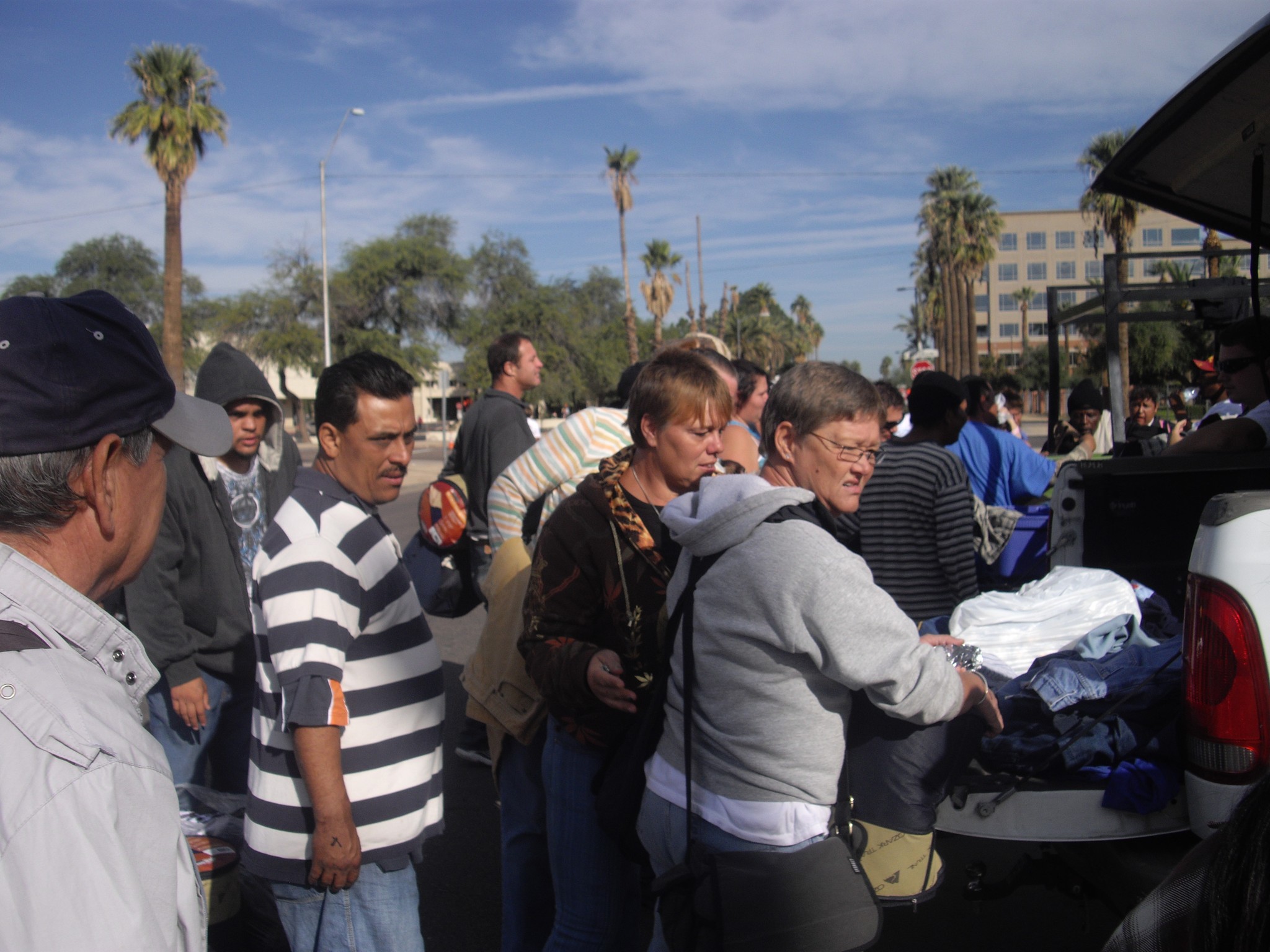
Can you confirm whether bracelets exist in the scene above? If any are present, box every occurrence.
[973,669,989,707]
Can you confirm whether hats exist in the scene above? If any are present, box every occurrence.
[0,289,234,457]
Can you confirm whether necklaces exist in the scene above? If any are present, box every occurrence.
[630,462,660,520]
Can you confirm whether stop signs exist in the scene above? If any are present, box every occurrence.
[908,362,936,380]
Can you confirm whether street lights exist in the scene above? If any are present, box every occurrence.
[320,108,366,367]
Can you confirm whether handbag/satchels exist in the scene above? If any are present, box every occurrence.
[594,689,665,867]
[657,833,885,952]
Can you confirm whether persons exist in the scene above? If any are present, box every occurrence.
[943,373,1096,510]
[856,371,980,621]
[241,349,450,950]
[873,379,905,444]
[517,350,744,952]
[488,344,741,950]
[121,340,303,814]
[636,361,1002,952]
[433,334,546,766]
[714,358,771,477]
[992,341,1243,459]
[0,290,209,951]
[1156,314,1269,456]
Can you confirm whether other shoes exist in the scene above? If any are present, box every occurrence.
[455,720,492,766]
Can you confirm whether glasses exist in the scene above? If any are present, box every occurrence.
[808,431,887,466]
